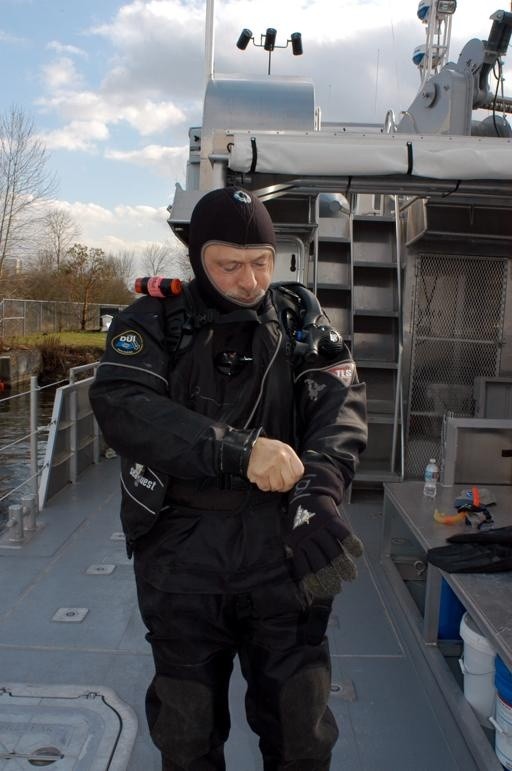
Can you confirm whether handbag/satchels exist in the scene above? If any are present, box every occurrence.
[415,525,510,577]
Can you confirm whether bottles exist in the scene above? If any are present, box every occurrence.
[424,458,439,499]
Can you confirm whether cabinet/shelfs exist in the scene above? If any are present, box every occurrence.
[377,482,512,771]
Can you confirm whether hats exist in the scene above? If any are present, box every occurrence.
[188,186,277,314]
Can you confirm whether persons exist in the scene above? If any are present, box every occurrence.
[88,187,367,770]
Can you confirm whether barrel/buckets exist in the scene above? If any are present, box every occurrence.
[455,611,498,731]
[439,578,462,642]
[488,656,510,769]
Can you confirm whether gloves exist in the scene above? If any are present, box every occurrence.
[281,494,364,599]
